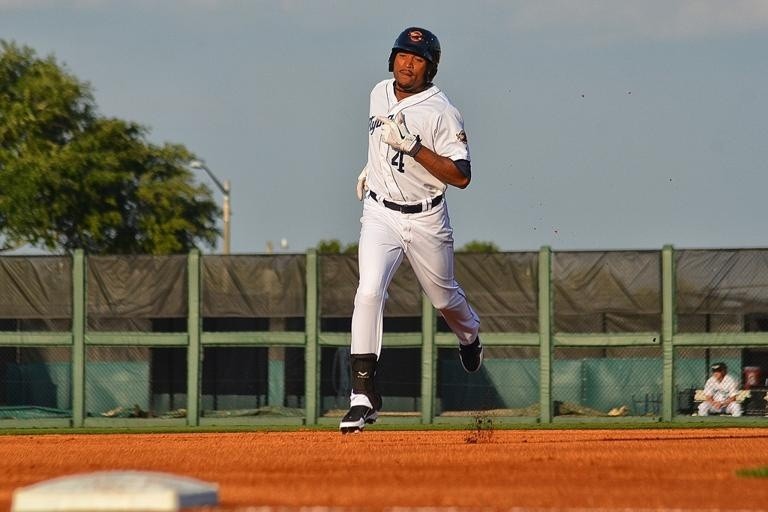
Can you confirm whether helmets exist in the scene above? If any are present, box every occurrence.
[388,27,441,83]
[712,363,726,372]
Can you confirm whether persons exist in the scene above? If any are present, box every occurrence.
[339,27,484,434]
[698,362,741,417]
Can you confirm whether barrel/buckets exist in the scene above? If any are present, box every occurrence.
[744,366,762,390]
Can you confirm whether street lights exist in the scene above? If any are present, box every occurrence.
[189,159,231,253]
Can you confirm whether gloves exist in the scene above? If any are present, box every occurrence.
[356,163,370,201]
[377,112,423,157]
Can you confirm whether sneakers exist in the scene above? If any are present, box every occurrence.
[340,392,383,432]
[458,335,483,373]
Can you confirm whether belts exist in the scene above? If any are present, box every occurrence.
[370,190,442,213]
